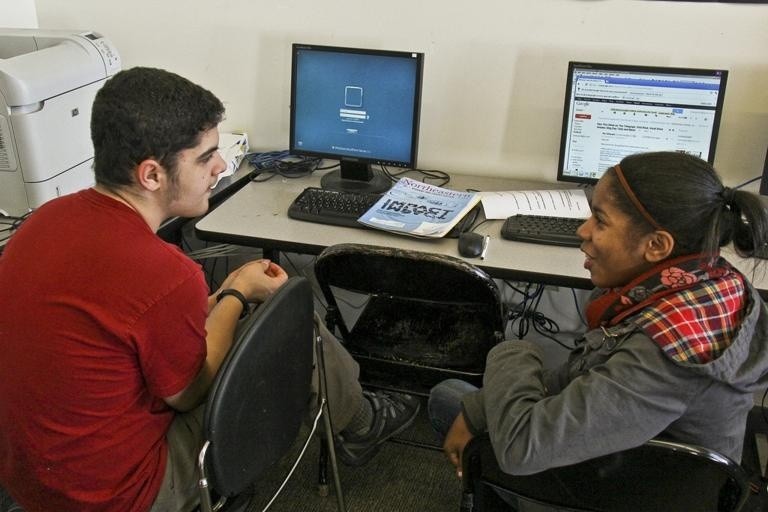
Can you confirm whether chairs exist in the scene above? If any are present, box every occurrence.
[177,273,350,511]
[311,241,511,497]
[458,425,752,510]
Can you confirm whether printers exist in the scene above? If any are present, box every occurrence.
[0,27,122,218]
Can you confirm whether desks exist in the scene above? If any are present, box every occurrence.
[0,151,265,254]
[188,155,768,316]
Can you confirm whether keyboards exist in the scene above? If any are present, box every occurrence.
[288,187,481,237]
[501,214,588,247]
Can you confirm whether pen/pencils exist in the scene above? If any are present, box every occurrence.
[480,235,491,261]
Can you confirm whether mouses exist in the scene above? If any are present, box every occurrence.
[459,232,484,258]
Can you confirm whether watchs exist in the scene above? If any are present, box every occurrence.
[216,288,249,320]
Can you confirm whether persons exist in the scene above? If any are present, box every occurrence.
[0,66,421,512]
[428,149,768,511]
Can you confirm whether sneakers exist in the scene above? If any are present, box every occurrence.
[335,386,422,467]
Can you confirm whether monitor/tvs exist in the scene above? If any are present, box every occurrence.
[558,61,729,185]
[289,44,424,192]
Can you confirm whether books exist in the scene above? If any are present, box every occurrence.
[357,175,484,241]
